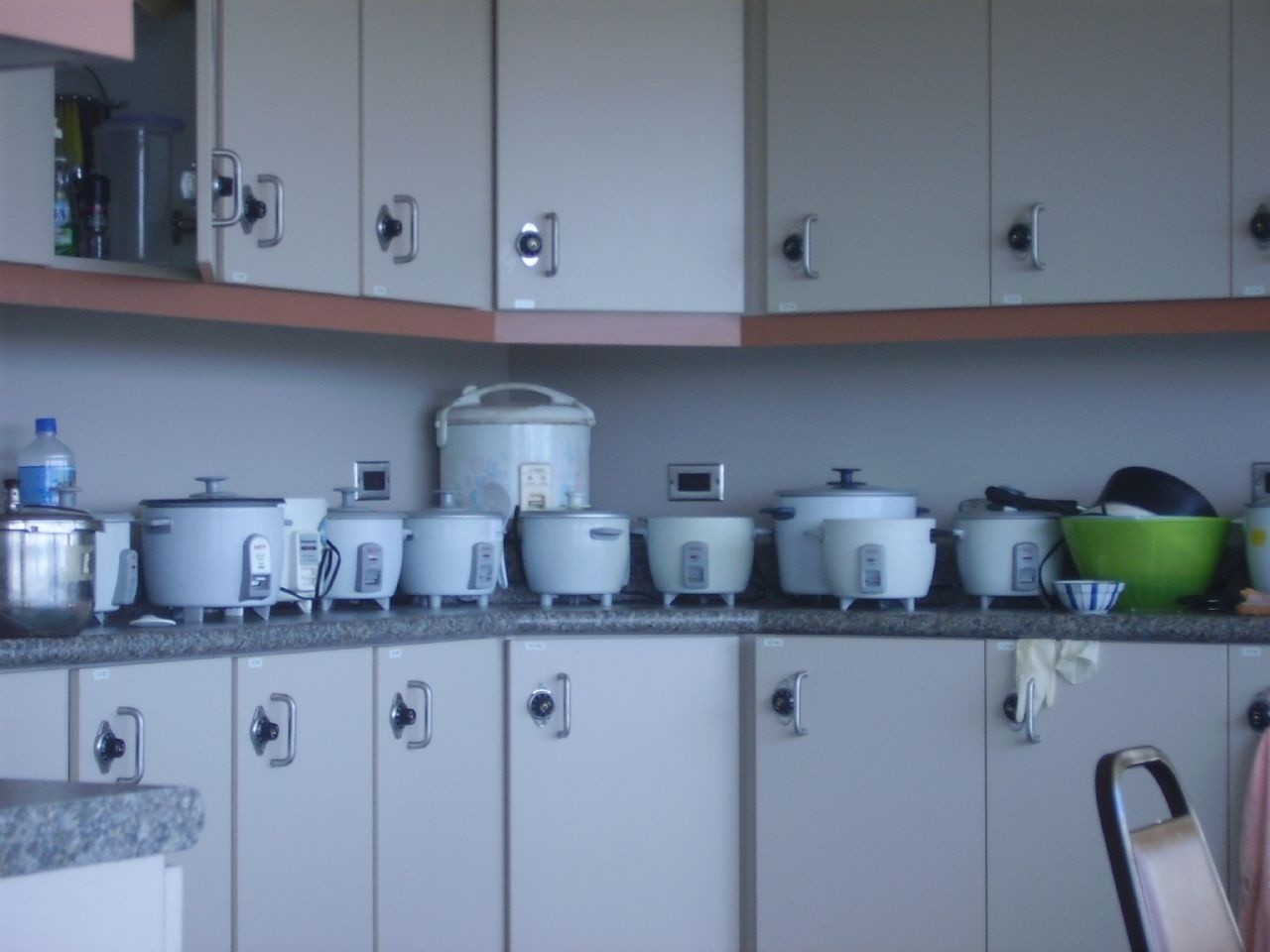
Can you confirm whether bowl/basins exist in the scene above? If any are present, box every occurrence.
[1058,513,1233,611]
[1053,578,1127,615]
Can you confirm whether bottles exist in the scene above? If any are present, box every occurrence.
[54,116,77,255]
[77,145,113,259]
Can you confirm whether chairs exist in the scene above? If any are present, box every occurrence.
[1098,746,1257,952]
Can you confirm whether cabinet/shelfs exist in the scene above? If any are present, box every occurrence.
[487,0,758,350]
[1,639,743,950]
[190,5,501,342]
[735,629,1263,952]
[750,2,1270,336]
[0,1,222,329]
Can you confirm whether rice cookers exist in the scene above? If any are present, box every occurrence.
[0,378,1081,635]
[1244,472,1269,595]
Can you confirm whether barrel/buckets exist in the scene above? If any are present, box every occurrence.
[94,122,172,263]
